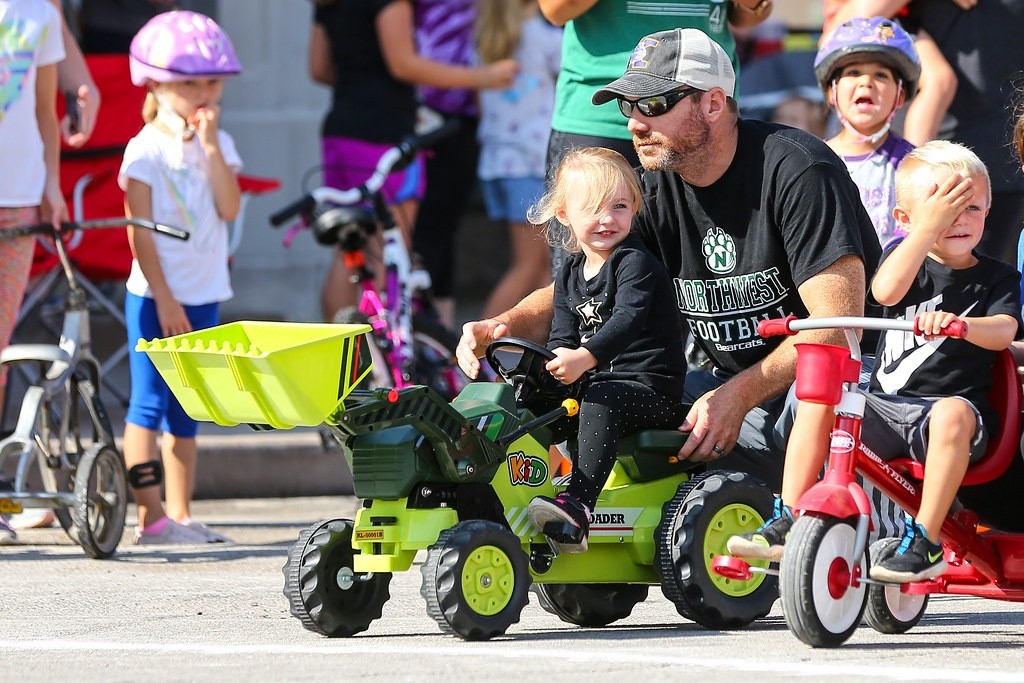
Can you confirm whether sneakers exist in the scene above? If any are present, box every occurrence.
[869,517,948,583]
[527,491,589,554]
[725,498,795,562]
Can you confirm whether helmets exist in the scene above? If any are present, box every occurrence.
[812,15,922,100]
[126,9,243,88]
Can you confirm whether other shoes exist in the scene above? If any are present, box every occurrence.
[185,523,234,543]
[133,519,205,544]
[1,511,18,545]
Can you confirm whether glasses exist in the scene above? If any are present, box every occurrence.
[615,85,698,118]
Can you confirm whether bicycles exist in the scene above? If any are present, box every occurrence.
[267,133,493,412]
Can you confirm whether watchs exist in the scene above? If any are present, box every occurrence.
[737,0,770,16]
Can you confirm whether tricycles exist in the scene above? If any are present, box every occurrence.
[0,219,190,560]
[754,312,1024,646]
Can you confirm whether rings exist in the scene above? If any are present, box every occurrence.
[713,444,724,456]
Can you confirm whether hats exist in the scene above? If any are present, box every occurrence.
[591,27,735,105]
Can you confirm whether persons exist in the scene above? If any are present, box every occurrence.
[116,11,245,544]
[453,27,878,464]
[530,147,678,554]
[309,0,1024,374]
[726,139,1021,583]
[0,0,100,416]
